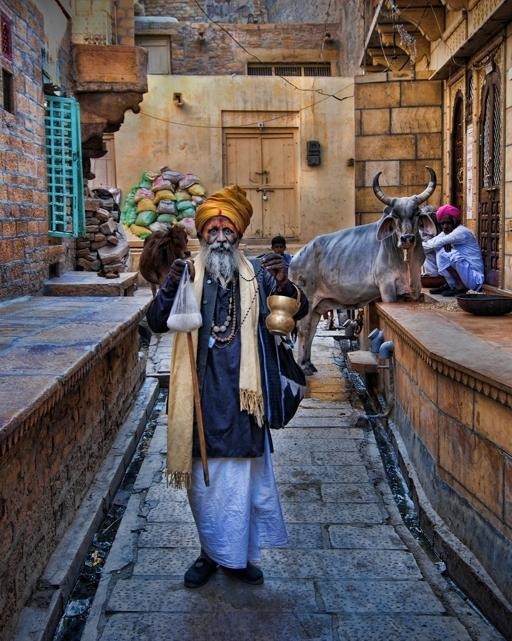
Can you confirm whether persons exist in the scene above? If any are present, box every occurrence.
[145,183,309,588]
[422,205,485,298]
[270,236,294,266]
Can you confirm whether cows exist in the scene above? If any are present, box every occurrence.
[138,222,192,300]
[286,166,437,376]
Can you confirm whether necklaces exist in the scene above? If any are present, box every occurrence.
[209,265,265,350]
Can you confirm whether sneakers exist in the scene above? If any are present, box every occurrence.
[219,559,265,586]
[429,283,467,296]
[182,551,220,589]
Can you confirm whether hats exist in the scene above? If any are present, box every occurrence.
[435,204,461,222]
[193,184,255,238]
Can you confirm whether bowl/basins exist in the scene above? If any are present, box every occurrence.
[421,273,446,289]
[456,291,512,316]
